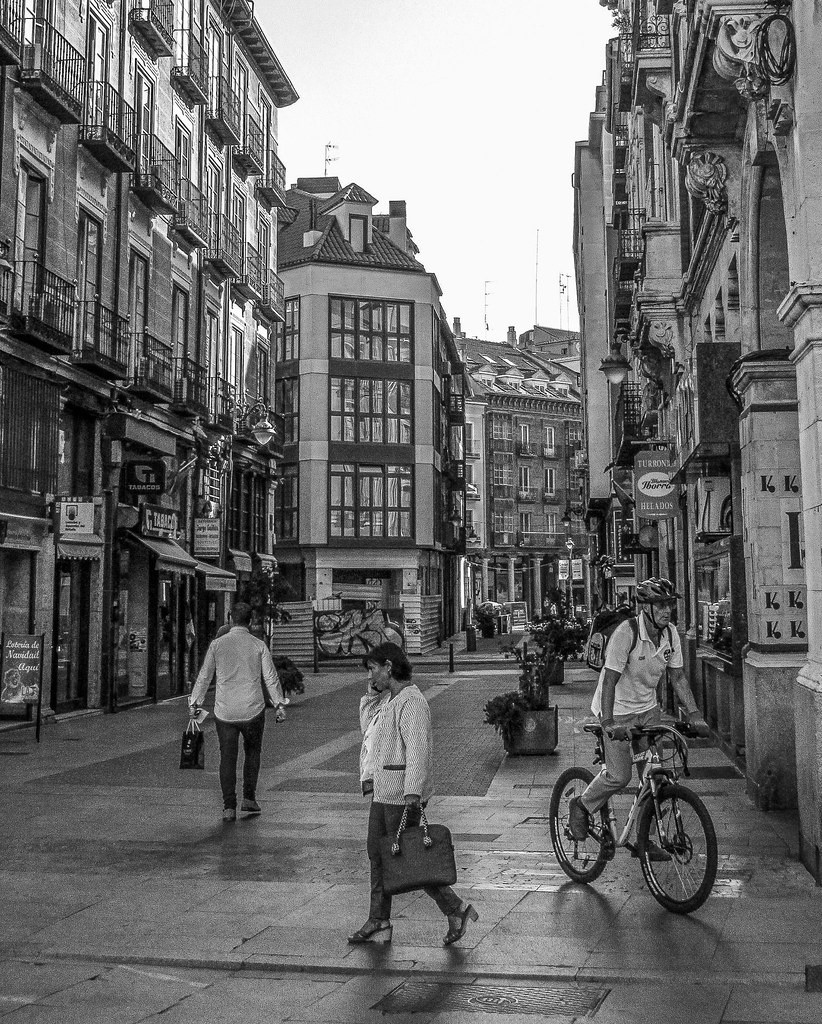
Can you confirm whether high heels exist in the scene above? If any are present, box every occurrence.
[348,917,393,943]
[443,904,479,944]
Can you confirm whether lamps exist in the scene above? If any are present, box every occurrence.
[598,327,639,384]
[236,394,277,445]
[560,506,585,526]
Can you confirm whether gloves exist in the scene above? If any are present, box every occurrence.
[601,718,624,742]
[689,711,710,738]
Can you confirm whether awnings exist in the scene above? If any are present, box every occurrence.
[128,533,198,577]
[195,559,236,592]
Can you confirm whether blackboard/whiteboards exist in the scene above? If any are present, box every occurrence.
[0,631,45,705]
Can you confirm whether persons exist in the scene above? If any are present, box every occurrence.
[187,603,290,823]
[346,641,479,944]
[568,577,710,862]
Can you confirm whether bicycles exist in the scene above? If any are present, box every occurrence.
[549,722,720,915]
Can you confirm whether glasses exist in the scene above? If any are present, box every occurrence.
[654,604,677,610]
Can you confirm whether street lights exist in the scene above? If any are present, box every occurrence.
[565,538,575,617]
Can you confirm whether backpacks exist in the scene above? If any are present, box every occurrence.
[586,604,674,672]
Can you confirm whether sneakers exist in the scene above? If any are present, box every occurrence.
[569,796,589,841]
[240,799,262,811]
[222,804,237,822]
[631,839,672,861]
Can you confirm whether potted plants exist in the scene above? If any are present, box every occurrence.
[477,603,587,753]
[261,654,305,707]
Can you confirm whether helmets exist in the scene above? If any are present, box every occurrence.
[637,577,683,603]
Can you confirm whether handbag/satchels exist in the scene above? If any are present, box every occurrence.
[179,720,205,770]
[378,802,457,897]
